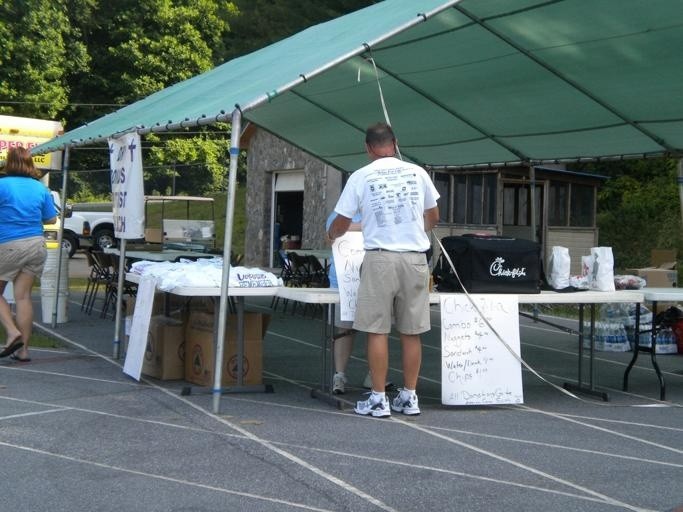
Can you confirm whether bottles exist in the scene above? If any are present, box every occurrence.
[584,302,679,355]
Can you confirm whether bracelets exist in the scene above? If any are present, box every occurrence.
[325,231,335,243]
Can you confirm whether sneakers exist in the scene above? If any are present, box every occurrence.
[353,374,393,417]
[391,388,421,415]
[332,372,346,394]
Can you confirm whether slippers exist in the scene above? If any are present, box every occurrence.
[0,342,31,361]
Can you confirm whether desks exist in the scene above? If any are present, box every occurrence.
[125,273,275,394]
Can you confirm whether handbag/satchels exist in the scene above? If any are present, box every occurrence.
[545,245,614,292]
[432,234,541,294]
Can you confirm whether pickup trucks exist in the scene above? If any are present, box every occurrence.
[45,191,145,258]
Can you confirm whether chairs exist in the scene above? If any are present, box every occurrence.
[80,245,330,320]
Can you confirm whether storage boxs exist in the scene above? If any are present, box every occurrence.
[628,249,679,313]
[123,295,272,389]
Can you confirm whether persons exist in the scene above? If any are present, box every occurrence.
[0,146,56,364]
[325,204,393,396]
[327,123,442,419]
